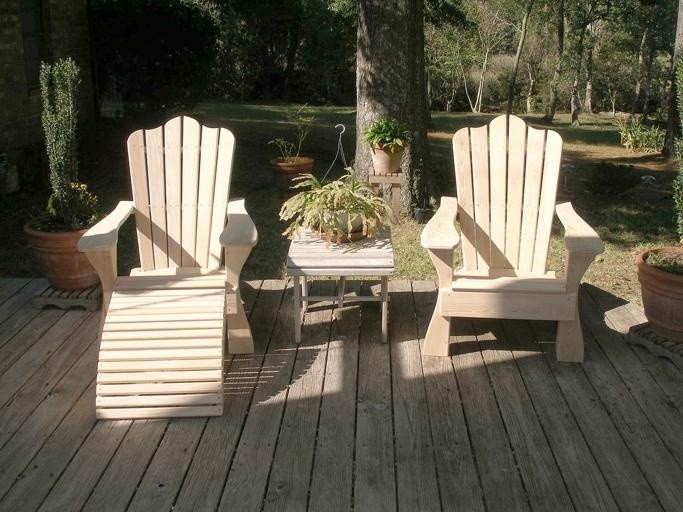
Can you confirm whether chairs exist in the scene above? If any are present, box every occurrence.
[78,115,260,420]
[419,114,604,364]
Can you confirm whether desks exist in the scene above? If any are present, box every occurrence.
[286,222,394,344]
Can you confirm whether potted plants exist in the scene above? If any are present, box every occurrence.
[23,56,109,291]
[266,102,316,191]
[360,116,412,175]
[277,166,392,255]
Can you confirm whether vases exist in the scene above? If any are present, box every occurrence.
[636,245,683,343]
